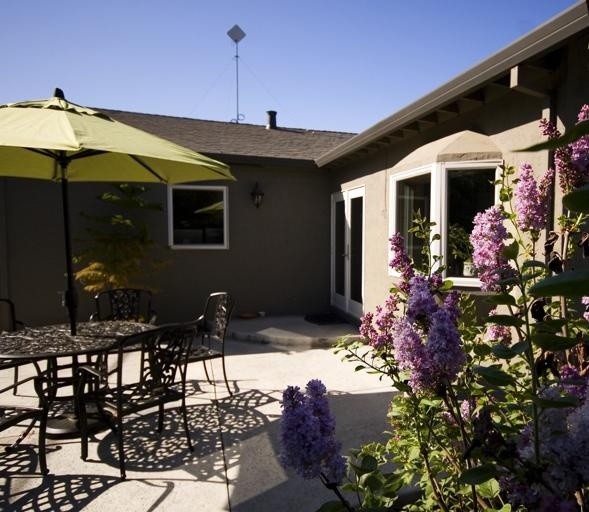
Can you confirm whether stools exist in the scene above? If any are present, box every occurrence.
[0,404,50,483]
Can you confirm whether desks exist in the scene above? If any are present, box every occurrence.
[0,322,159,440]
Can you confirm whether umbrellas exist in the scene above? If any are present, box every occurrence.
[0,88,239,420]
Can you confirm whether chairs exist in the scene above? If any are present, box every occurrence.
[0,297,51,396]
[157,290,236,396]
[71,322,201,484]
[91,287,154,397]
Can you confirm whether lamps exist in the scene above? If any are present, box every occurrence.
[251,179,266,208]
